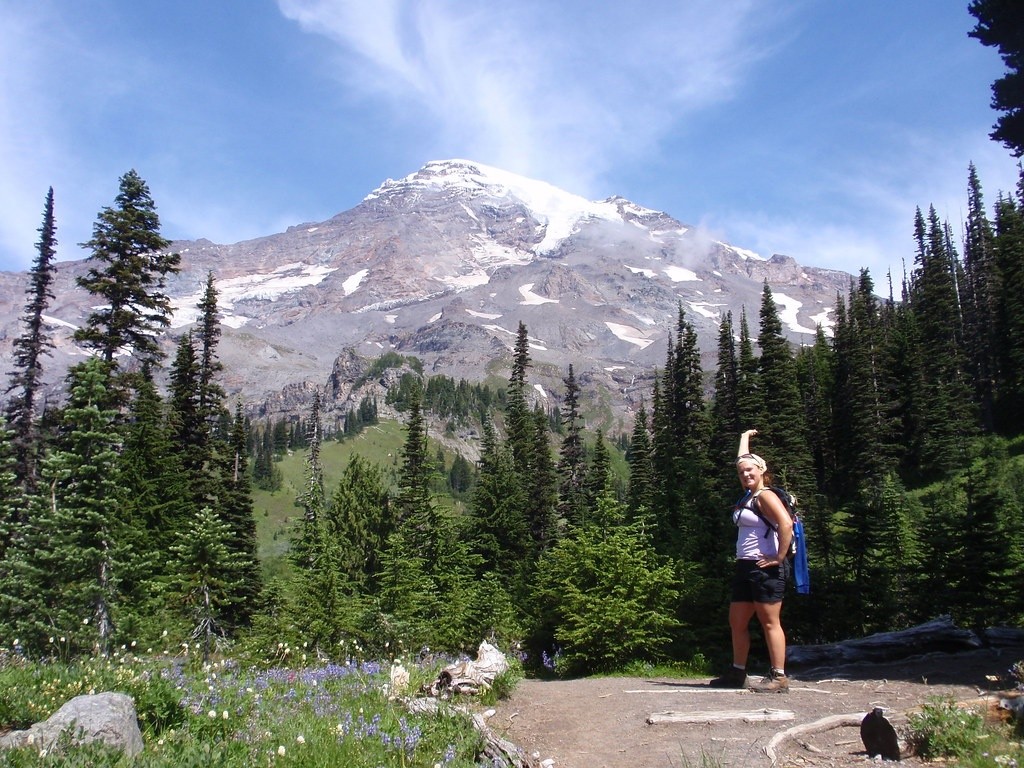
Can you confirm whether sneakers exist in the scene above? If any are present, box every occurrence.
[710,666,748,687]
[751,668,788,694]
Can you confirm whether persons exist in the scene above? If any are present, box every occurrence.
[710,429,793,694]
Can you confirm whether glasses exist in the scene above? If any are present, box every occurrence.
[735,454,760,463]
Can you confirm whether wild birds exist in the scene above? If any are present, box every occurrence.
[859,707,901,762]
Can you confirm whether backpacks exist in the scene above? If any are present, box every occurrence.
[738,486,798,560]
